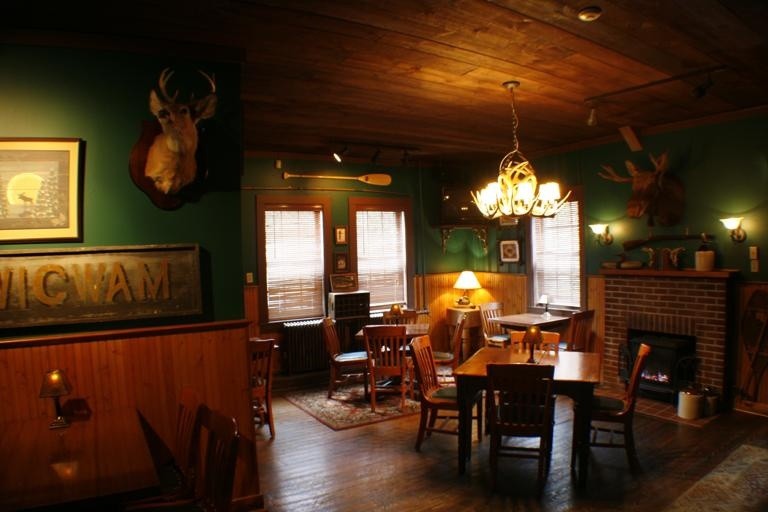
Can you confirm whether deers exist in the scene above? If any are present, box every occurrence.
[144,64,219,197]
[596,150,684,228]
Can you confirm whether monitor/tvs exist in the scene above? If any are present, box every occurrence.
[441,185,487,224]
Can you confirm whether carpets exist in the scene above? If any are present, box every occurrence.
[661,443,767,511]
[285,367,498,433]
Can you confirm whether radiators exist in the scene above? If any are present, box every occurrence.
[282,313,383,377]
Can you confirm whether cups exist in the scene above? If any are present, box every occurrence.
[658,248,671,272]
[693,250,715,272]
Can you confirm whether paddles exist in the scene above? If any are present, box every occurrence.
[284,172,392,186]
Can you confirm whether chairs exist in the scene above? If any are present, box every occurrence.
[479,301,510,349]
[509,330,560,352]
[247,337,276,441]
[125,399,243,512]
[559,308,596,352]
[571,342,651,477]
[321,317,371,401]
[433,315,466,384]
[382,310,417,325]
[483,363,557,493]
[361,325,414,414]
[408,334,483,461]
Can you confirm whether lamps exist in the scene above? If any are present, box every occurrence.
[717,215,747,244]
[453,269,479,305]
[468,78,574,220]
[587,222,613,246]
[37,364,74,432]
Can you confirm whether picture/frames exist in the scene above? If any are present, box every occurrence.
[332,251,351,273]
[0,135,86,246]
[494,238,522,266]
[334,224,348,246]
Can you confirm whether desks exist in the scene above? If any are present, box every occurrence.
[487,312,568,330]
[451,346,602,479]
[356,324,430,397]
[0,405,163,512]
